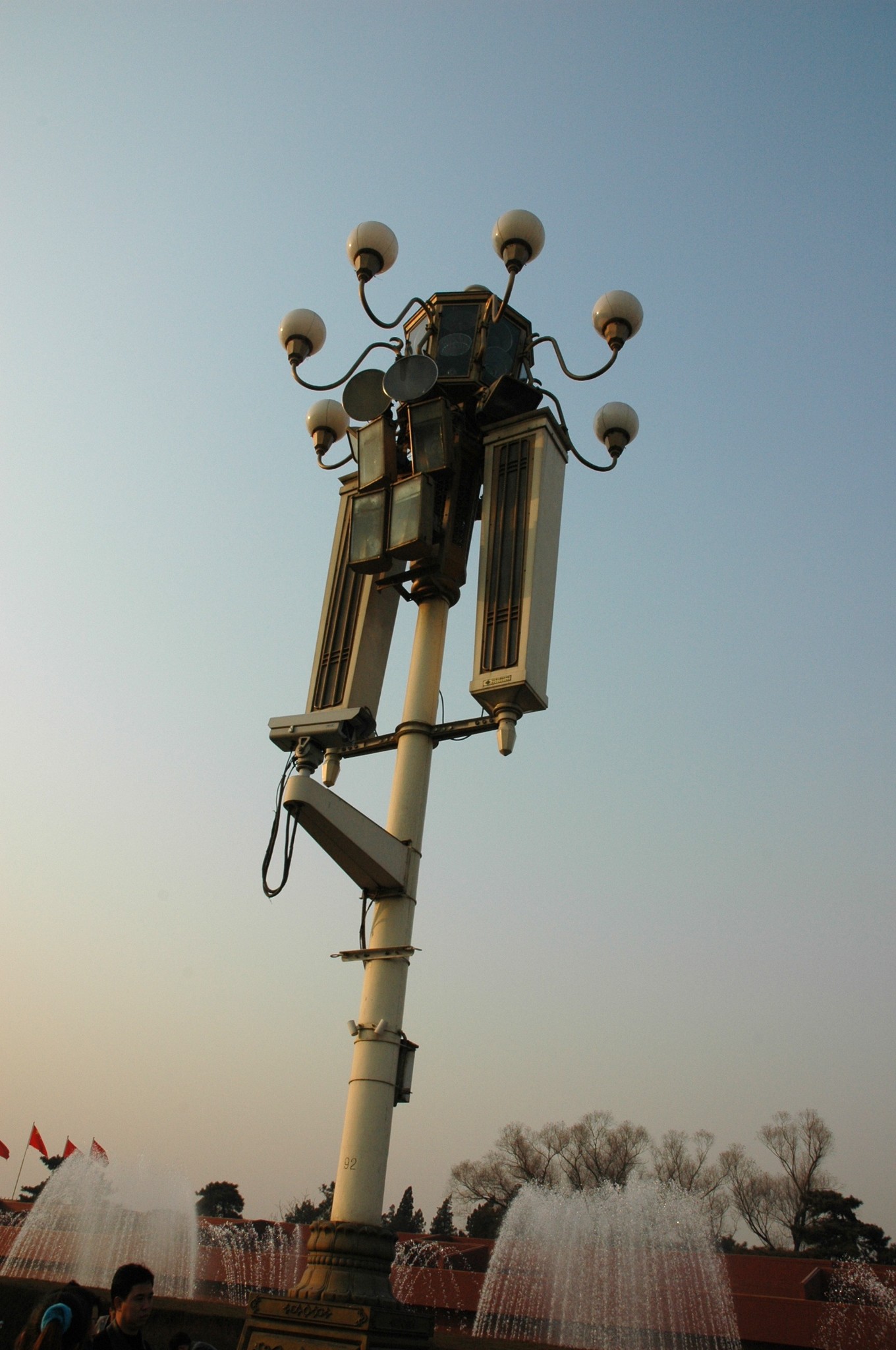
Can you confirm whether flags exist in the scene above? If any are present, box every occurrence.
[28,1125,48,1157]
[0,1140,10,1160]
[64,1140,85,1159]
[89,1140,109,1167]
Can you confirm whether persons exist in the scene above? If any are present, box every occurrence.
[169,1332,192,1350]
[95,1302,116,1334]
[15,1280,101,1350]
[90,1264,155,1350]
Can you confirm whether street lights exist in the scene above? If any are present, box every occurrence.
[239,208,639,1350]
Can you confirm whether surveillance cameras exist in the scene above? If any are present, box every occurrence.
[265,706,376,752]
